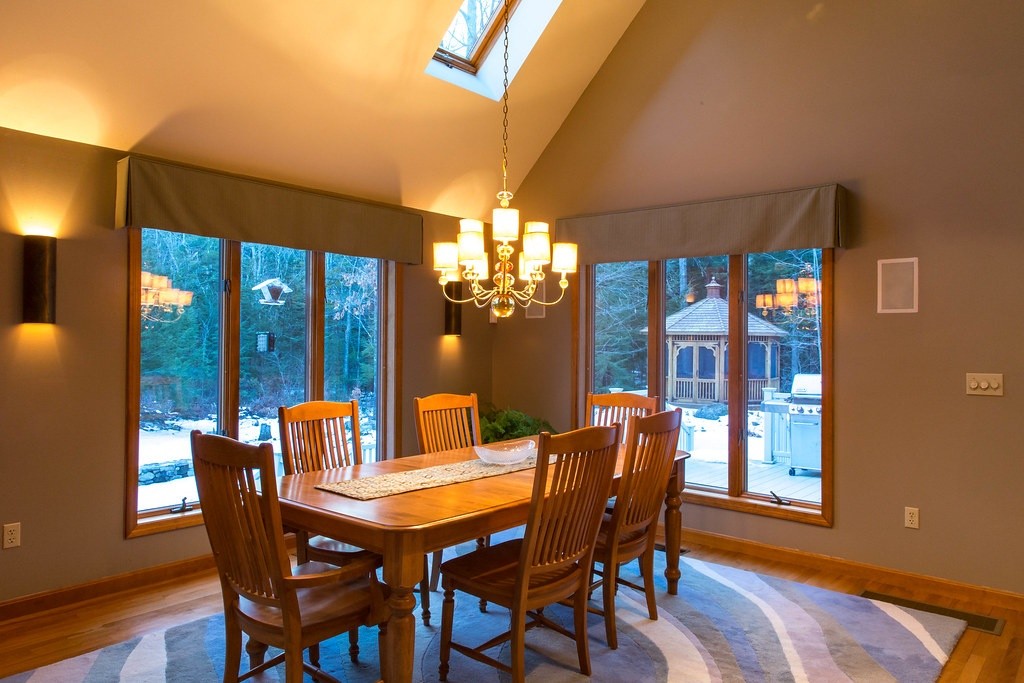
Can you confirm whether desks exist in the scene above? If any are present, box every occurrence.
[238,435,690,683]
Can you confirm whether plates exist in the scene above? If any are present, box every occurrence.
[473,439,536,465]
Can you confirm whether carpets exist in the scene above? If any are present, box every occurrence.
[0,525,967,683]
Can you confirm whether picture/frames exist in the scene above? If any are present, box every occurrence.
[876,257,919,313]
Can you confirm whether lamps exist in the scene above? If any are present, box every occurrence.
[756,262,823,331]
[433,0,577,318]
[686,286,695,302]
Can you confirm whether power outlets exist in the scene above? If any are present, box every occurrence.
[3,522,21,549]
[905,507,920,529]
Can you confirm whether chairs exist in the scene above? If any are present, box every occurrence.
[412,393,492,616]
[534,407,683,650]
[191,429,392,683]
[439,421,622,683]
[584,391,660,596]
[277,398,430,663]
